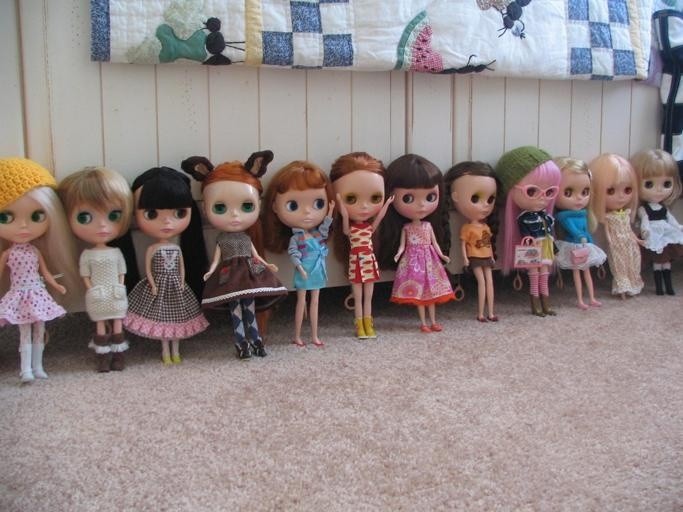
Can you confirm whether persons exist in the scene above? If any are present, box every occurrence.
[329,152,395,339]
[0,157,81,385]
[121,168,209,365]
[373,155,439,326]
[182,149,281,360]
[264,162,335,345]
[497,146,557,317]
[551,157,602,306]
[630,149,671,290]
[58,166,136,373]
[443,161,495,320]
[589,154,642,294]
